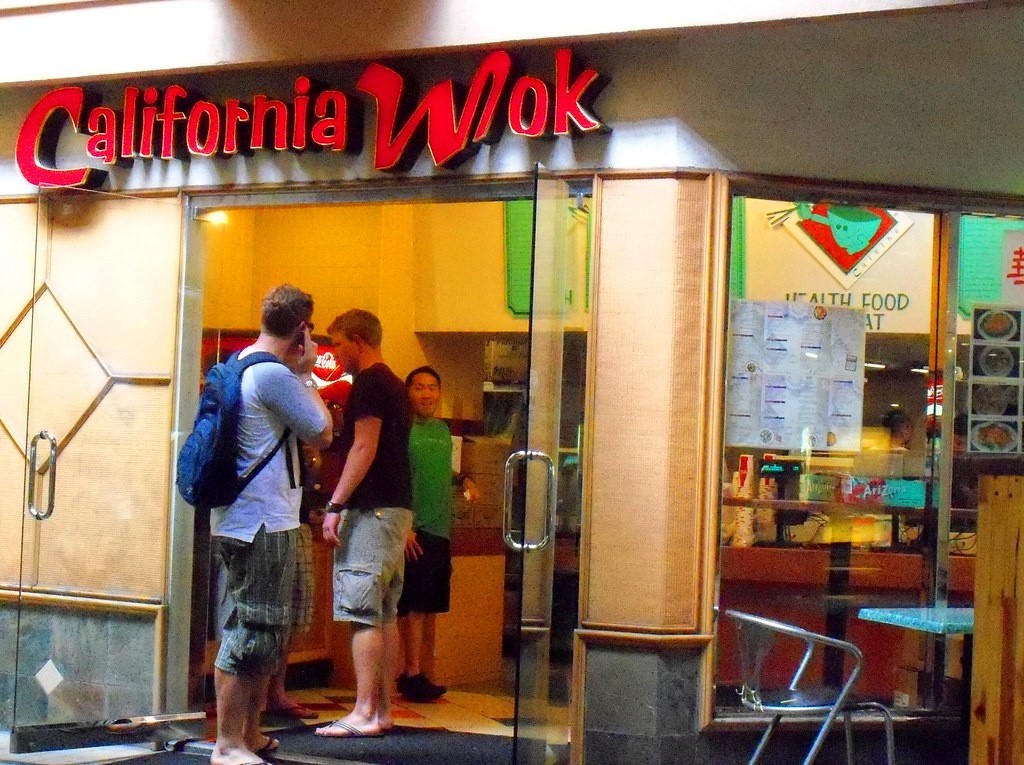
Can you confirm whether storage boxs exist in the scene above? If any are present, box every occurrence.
[450,436,513,528]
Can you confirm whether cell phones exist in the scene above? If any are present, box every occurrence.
[296,328,305,346]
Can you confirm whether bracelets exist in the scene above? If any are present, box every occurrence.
[454,472,473,489]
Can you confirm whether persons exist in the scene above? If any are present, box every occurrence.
[316,308,414,736]
[395,365,480,701]
[882,408,917,451]
[951,409,979,532]
[209,282,333,765]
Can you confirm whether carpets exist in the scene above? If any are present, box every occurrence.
[214,722,546,765]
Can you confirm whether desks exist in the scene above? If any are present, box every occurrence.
[858,606,974,765]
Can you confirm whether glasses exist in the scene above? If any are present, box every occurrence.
[305,321,314,331]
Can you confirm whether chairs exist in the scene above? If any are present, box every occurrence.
[726,610,897,765]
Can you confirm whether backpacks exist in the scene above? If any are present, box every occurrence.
[176,350,307,510]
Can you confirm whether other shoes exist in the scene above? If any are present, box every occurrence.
[396,673,447,700]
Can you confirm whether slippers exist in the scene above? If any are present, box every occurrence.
[312,719,397,738]
[210,759,278,765]
[266,704,319,719]
[249,736,279,753]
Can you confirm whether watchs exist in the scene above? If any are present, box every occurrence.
[325,501,344,513]
[304,379,317,389]
